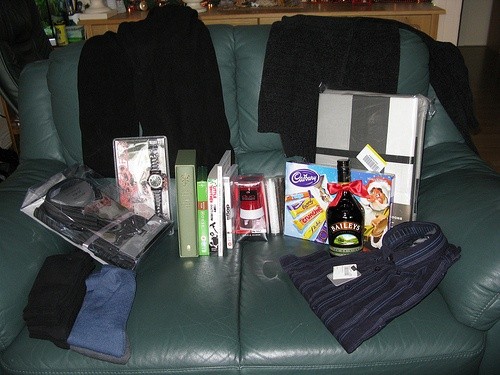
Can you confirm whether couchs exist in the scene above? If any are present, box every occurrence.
[0,4,500,375]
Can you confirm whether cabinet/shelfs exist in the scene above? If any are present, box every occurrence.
[258,4,445,41]
[76,5,258,39]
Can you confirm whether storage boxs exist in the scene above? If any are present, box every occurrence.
[280,159,394,252]
[174,149,200,258]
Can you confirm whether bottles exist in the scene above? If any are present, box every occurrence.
[326,159,365,257]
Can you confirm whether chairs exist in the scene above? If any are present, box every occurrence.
[0,0,55,116]
